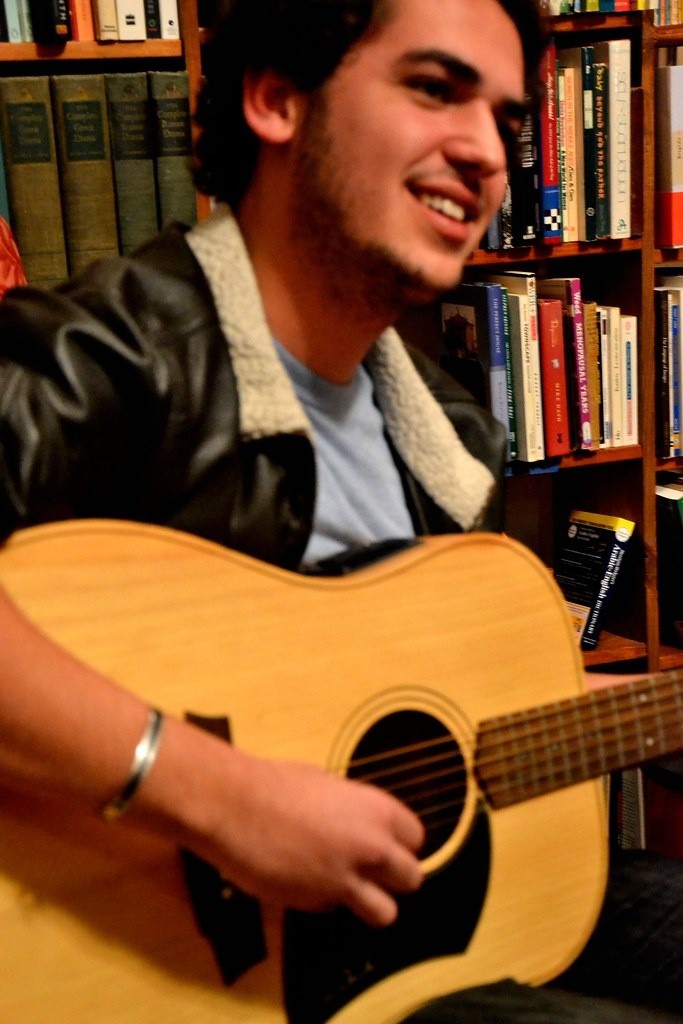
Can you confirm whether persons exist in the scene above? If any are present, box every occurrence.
[0,0,683,1024]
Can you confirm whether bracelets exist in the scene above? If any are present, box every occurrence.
[99,706,166,819]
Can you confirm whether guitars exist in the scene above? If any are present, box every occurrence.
[1,514,682,1023]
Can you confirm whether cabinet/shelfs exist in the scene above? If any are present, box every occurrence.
[3,0,681,854]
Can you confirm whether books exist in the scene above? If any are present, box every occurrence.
[2,3,680,666]
